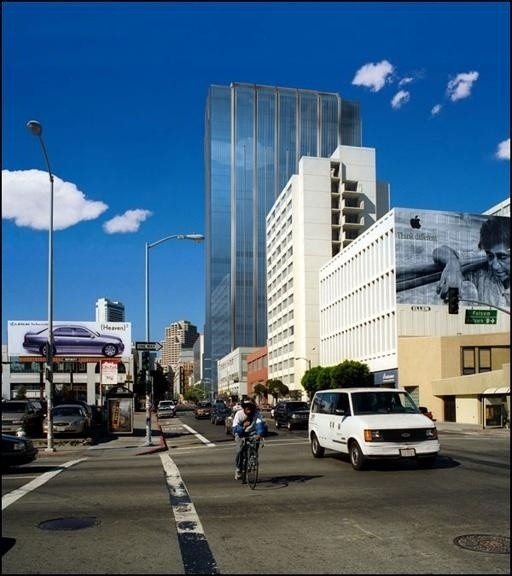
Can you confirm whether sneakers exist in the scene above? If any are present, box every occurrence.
[235,468,243,479]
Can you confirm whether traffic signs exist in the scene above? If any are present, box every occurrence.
[135,341,164,353]
[464,308,497,325]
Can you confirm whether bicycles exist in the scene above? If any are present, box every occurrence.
[235,434,264,490]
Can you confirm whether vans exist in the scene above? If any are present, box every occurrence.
[308,386,441,471]
[158,400,177,416]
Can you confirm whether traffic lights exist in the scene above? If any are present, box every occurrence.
[448,286,460,315]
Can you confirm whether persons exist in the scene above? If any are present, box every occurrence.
[432,216,511,308]
[231,399,265,480]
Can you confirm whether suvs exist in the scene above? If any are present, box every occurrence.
[273,400,310,432]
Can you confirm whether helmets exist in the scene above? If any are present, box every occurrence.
[242,397,257,408]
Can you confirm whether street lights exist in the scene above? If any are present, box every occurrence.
[144,231,206,447]
[26,119,56,454]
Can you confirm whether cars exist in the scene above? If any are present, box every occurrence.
[22,323,125,358]
[0,398,42,437]
[0,431,40,469]
[194,400,270,439]
[158,405,175,419]
[40,403,93,438]
